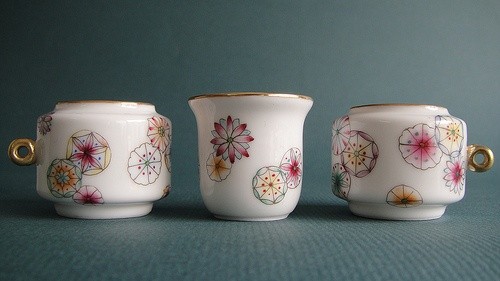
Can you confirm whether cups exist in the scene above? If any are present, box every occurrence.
[187,91,314,222]
[8,100,172,219]
[330,103,495,221]
